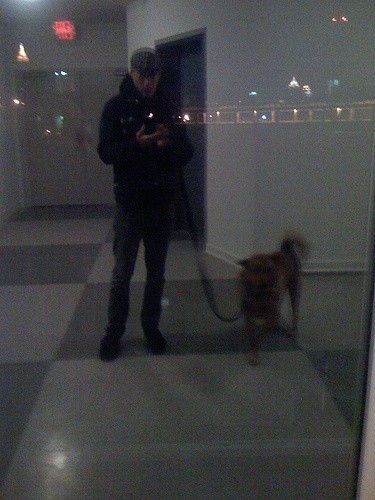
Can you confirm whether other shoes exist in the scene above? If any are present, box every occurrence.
[99,334,122,361]
[145,331,168,353]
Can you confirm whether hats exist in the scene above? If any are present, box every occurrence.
[130,51,163,75]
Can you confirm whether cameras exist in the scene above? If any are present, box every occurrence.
[141,110,157,135]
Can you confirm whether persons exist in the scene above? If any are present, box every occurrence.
[97,47,195,362]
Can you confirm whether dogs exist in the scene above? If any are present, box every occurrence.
[234,234,311,367]
